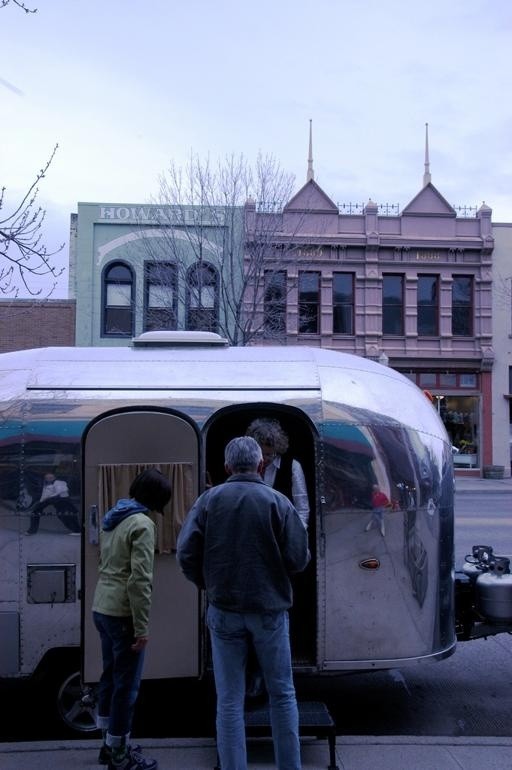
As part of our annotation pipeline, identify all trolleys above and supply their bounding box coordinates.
[397,479,431,606]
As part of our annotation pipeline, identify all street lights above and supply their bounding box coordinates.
[378,348,390,368]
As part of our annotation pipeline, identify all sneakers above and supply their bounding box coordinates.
[98,738,159,770]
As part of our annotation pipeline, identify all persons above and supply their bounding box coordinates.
[246,418,311,534]
[176,435,324,770]
[26,472,82,537]
[365,485,389,538]
[92,467,173,770]
